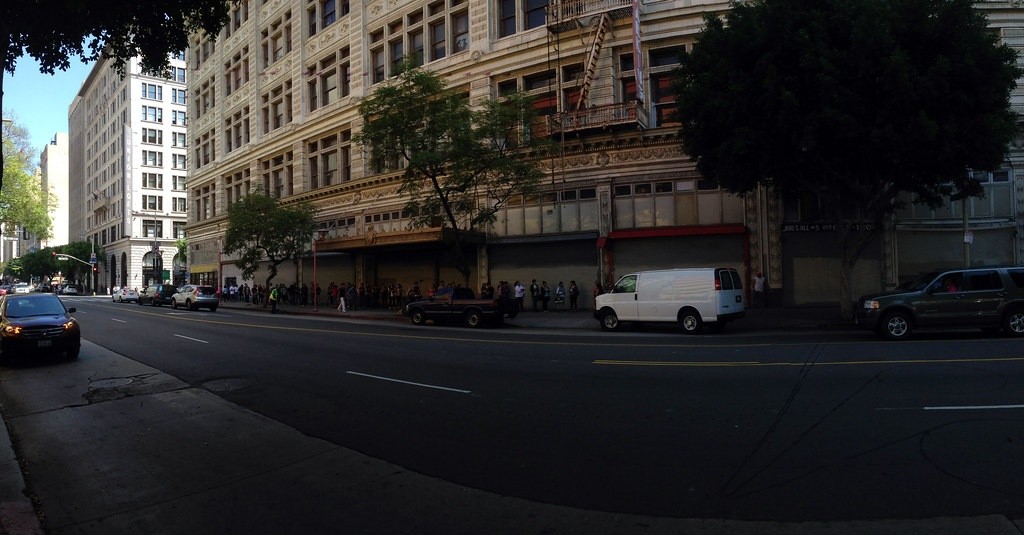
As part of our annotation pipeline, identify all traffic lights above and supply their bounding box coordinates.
[93,267,97,275]
[51,252,56,262]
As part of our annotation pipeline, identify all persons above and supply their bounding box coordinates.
[591,281,600,298]
[945,278,958,292]
[215,279,579,314]
[752,272,769,309]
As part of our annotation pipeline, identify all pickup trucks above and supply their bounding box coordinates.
[406,286,503,328]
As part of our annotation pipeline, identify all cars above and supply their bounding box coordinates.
[111,288,139,303]
[0,293,80,359]
[0,281,79,294]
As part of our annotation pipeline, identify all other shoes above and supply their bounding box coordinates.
[343,310,346,312]
[337,309,341,312]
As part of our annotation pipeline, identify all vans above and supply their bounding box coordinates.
[594,267,744,333]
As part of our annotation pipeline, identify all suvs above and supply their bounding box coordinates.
[848,264,1024,339]
[170,284,220,311]
[138,284,179,306]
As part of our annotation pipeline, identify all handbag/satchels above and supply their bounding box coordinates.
[554,294,563,303]
